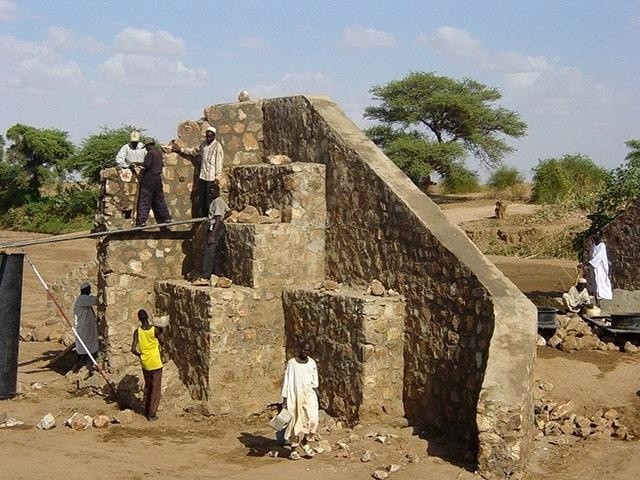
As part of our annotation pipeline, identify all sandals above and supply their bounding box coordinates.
[289,451,300,460]
[304,451,314,458]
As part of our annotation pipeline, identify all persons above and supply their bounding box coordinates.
[556,277,593,318]
[280,341,319,460]
[190,184,232,286]
[71,283,99,373]
[576,230,613,307]
[129,137,171,227]
[171,126,224,217]
[130,309,164,423]
[114,130,148,168]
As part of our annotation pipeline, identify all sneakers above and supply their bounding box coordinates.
[191,277,209,286]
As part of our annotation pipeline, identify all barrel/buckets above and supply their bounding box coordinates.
[587,306,601,316]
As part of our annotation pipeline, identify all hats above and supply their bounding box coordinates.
[577,277,589,283]
[129,131,139,142]
[80,282,91,290]
[205,126,217,135]
[141,137,155,150]
